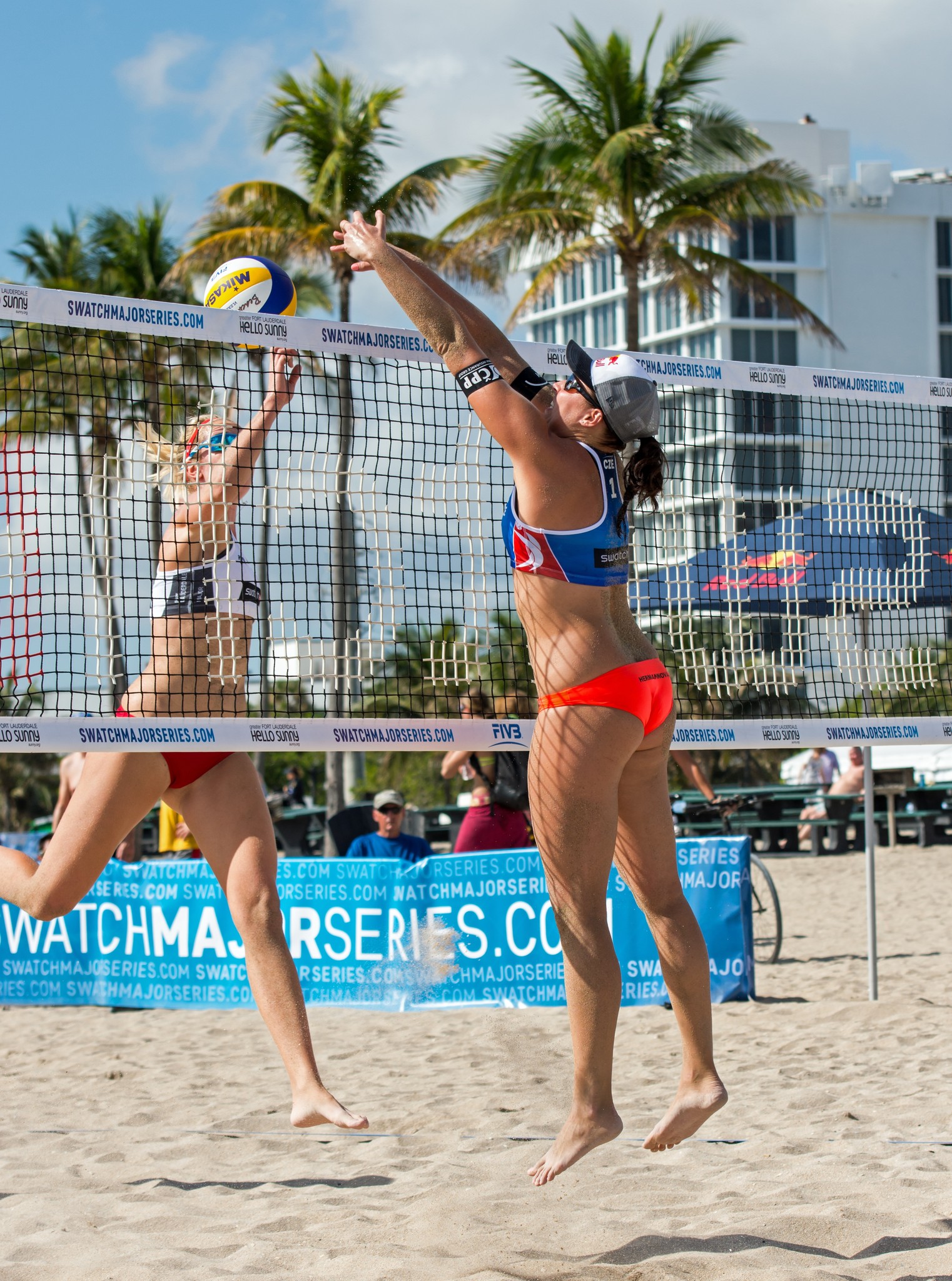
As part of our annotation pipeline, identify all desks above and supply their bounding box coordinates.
[764,795,860,855]
[681,795,742,802]
[273,805,325,858]
[672,787,822,792]
[415,805,469,846]
[873,784,952,811]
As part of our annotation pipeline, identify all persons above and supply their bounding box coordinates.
[670,750,737,818]
[37,833,54,860]
[0,347,367,1130]
[330,207,727,1188]
[782,745,866,850]
[158,799,203,860]
[441,686,539,854]
[279,764,435,864]
[52,712,136,862]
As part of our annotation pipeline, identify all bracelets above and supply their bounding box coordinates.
[710,796,717,804]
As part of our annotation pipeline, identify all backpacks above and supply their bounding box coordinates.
[467,751,529,817]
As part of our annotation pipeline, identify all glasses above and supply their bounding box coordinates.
[563,373,610,427]
[457,702,470,712]
[376,806,402,815]
[179,433,238,469]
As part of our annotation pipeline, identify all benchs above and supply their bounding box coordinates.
[681,810,947,848]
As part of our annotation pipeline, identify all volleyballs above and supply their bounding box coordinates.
[203,255,298,350]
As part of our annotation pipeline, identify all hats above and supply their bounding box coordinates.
[566,339,660,444]
[372,789,404,809]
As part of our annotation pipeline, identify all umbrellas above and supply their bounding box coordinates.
[627,488,952,1004]
